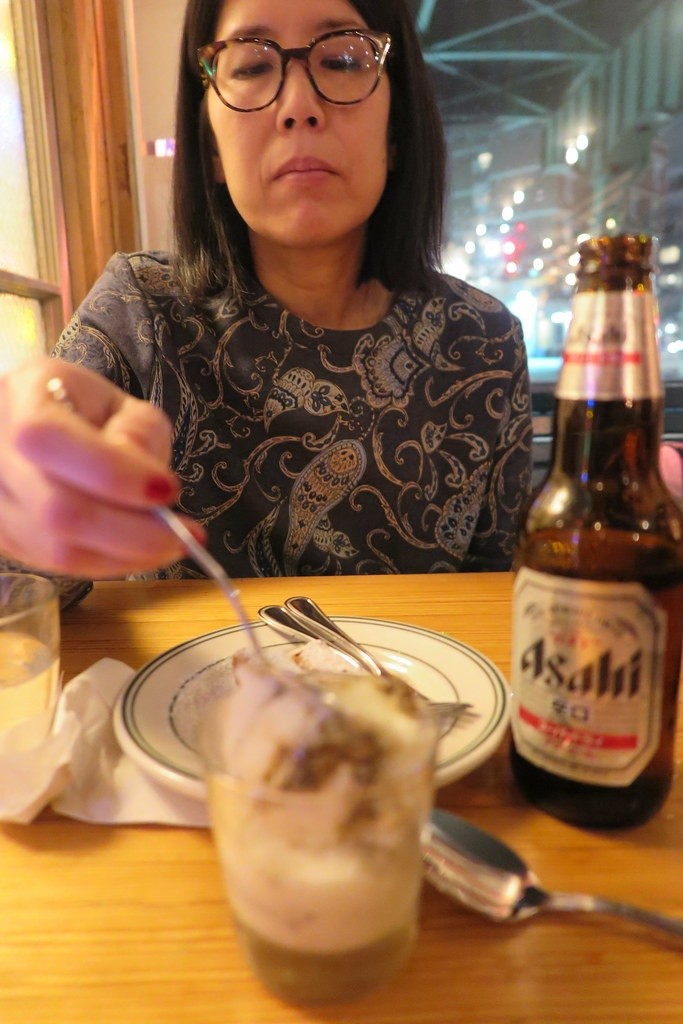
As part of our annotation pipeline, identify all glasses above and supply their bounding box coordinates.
[197,29,391,113]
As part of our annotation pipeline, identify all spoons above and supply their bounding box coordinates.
[418,807,683,938]
[48,376,355,733]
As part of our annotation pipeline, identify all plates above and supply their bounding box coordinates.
[111,617,511,800]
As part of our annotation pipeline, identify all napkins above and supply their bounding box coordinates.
[1,655,211,831]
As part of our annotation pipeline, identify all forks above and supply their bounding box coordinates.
[258,596,472,725]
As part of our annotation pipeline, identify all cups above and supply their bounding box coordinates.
[199,669,439,1011]
[0,572,61,751]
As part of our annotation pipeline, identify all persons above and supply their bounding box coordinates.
[0,1,532,613]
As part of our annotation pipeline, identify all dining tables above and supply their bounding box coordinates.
[1,570,683,1024]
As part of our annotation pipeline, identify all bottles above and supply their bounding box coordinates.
[506,229,683,834]
